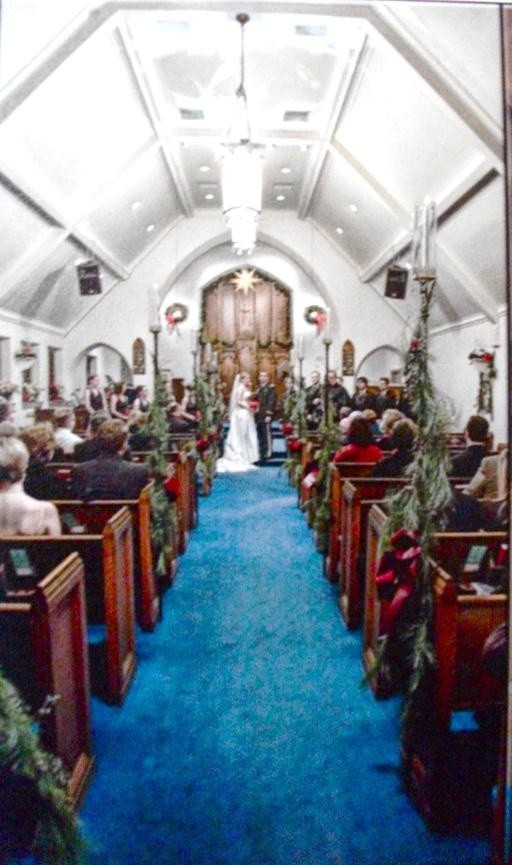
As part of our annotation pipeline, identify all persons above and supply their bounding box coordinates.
[253,371,276,459]
[213,372,260,473]
[1,436,61,535]
[283,371,507,534]
[1,370,227,464]
[18,428,72,500]
[70,419,149,500]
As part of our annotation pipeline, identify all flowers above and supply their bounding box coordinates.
[247,401,260,410]
[303,305,328,326]
[468,342,500,423]
[165,303,188,323]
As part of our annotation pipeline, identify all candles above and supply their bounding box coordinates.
[150,284,165,326]
[416,199,435,265]
[325,302,334,338]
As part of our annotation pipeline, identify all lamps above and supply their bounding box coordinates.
[209,13,271,257]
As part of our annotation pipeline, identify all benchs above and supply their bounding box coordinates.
[278,416,509,831]
[0,388,229,835]
